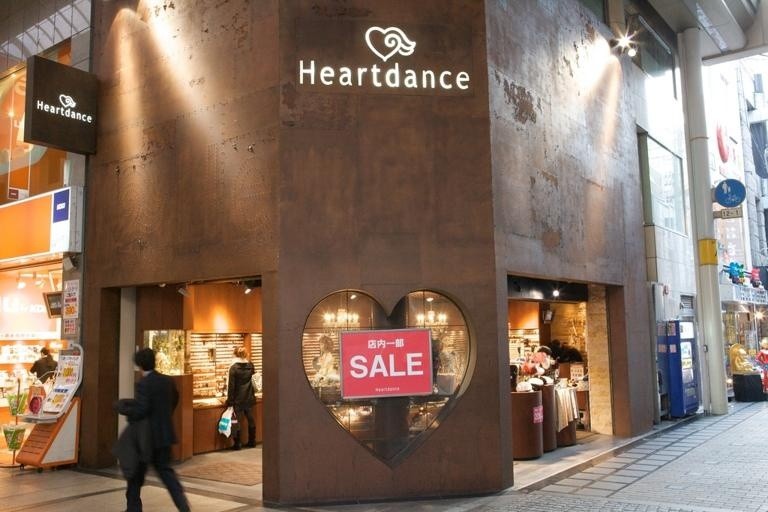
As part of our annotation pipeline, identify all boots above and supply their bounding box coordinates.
[232,429,241,450]
[242,426,256,448]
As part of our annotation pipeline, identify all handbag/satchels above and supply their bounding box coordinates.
[231,412,240,431]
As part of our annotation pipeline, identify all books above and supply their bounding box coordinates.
[39,383,77,414]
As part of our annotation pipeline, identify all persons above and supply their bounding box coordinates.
[756,336,768,393]
[309,333,340,386]
[734,348,756,373]
[108,345,198,512]
[436,332,461,372]
[29,395,43,414]
[223,344,260,451]
[28,346,59,384]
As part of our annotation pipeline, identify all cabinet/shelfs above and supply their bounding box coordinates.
[531,383,555,453]
[510,390,543,461]
[555,385,577,448]
[0,338,60,432]
[192,391,263,456]
